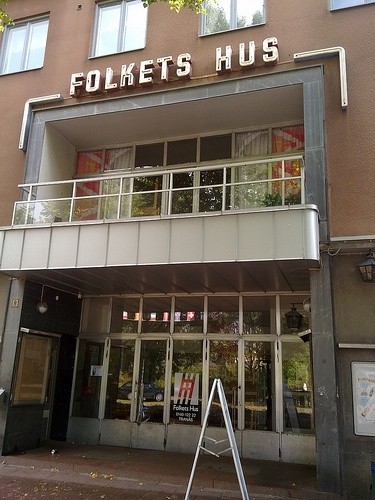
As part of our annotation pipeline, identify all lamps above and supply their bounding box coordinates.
[355,248,375,283]
[284,303,303,330]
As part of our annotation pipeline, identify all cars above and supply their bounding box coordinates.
[116,383,165,402]
